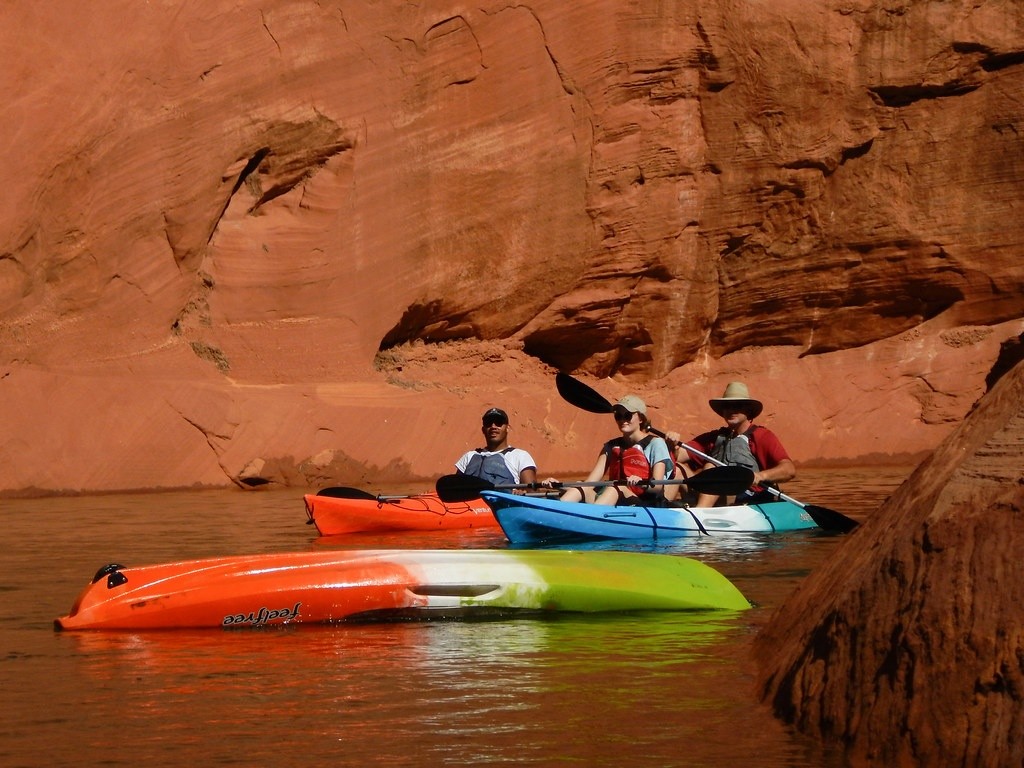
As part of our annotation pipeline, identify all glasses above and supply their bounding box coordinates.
[614,411,636,422]
[483,416,508,427]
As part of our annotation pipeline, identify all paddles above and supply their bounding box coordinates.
[436,465,755,503]
[316,486,527,500]
[555,372,860,534]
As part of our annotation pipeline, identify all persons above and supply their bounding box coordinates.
[665,382,796,508]
[540,395,674,506]
[455,408,538,496]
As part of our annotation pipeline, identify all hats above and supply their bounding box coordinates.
[612,394,647,413]
[709,381,763,420]
[482,407,508,423]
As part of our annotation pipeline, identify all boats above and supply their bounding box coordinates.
[299,493,530,536]
[54,550,756,627]
[478,489,821,545]
